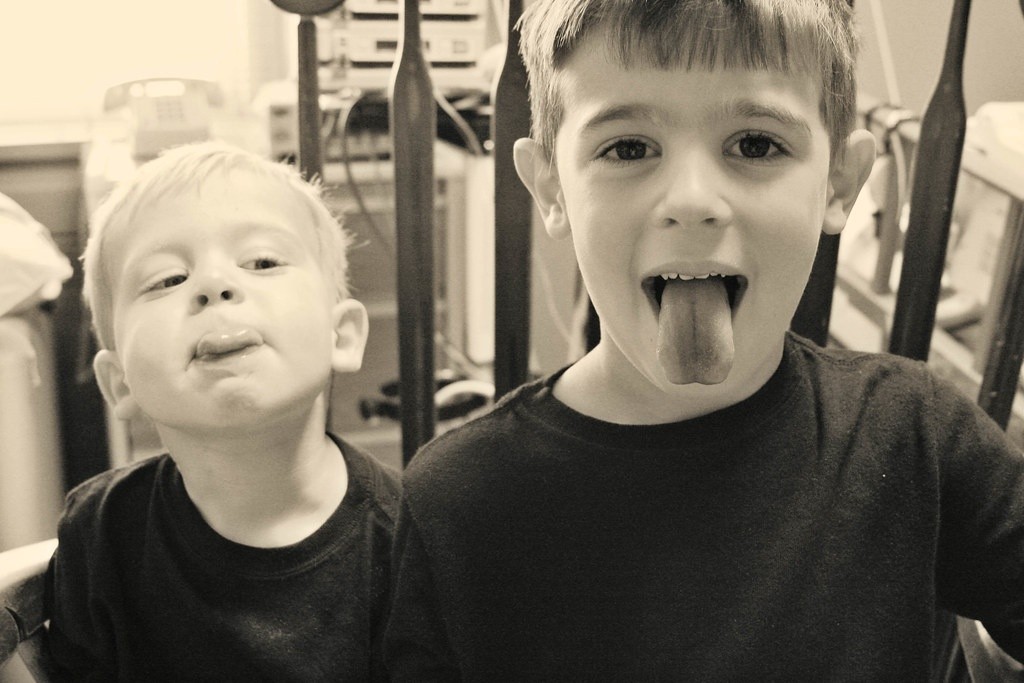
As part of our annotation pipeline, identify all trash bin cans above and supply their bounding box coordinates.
[355,368,498,470]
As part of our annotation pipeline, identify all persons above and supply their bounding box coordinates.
[44,146,403,682]
[380,0,1023,683]
